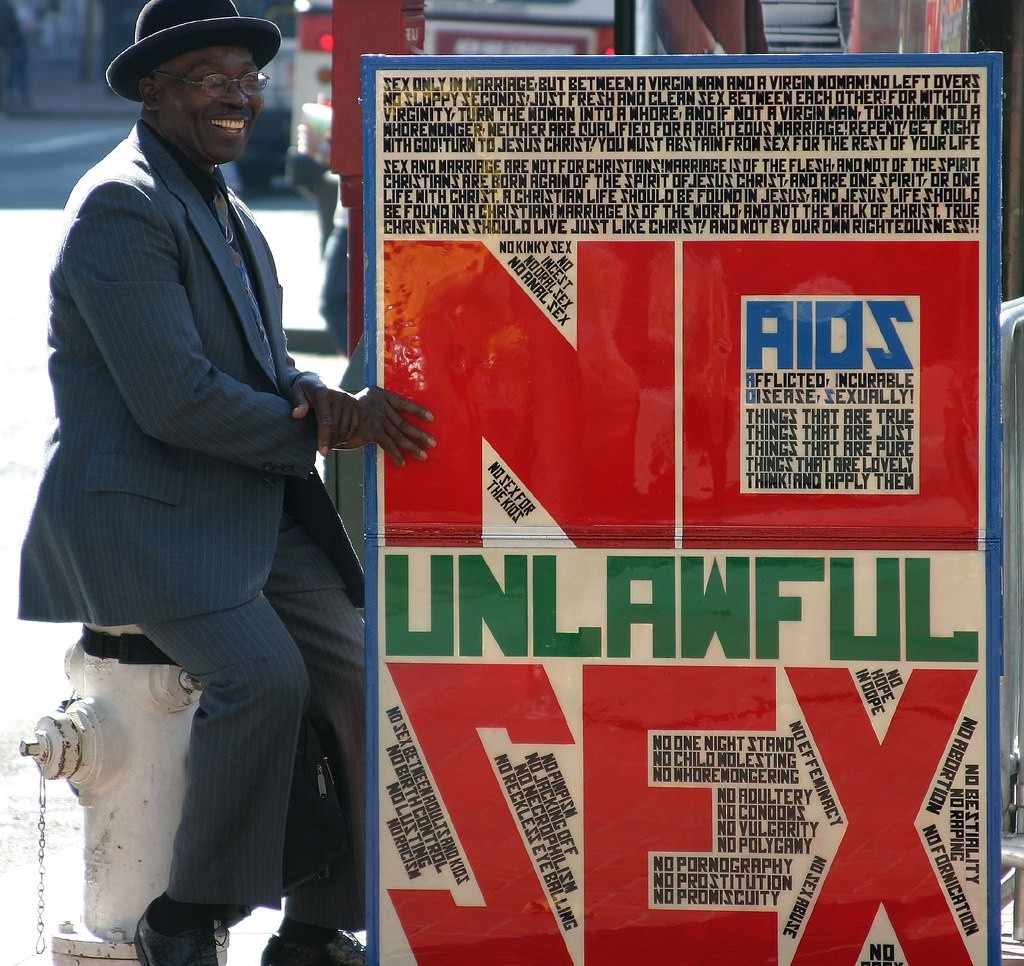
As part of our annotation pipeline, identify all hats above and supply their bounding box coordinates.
[106,0,282,103]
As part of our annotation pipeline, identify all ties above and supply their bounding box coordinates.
[213,184,276,381]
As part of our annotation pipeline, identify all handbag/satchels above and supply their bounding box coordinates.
[214,712,350,935]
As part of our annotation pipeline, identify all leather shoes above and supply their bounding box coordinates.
[134,896,229,966]
[261,932,365,966]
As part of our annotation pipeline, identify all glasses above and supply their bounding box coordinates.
[153,72,270,98]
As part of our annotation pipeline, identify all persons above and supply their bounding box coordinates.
[18,0,436,966]
